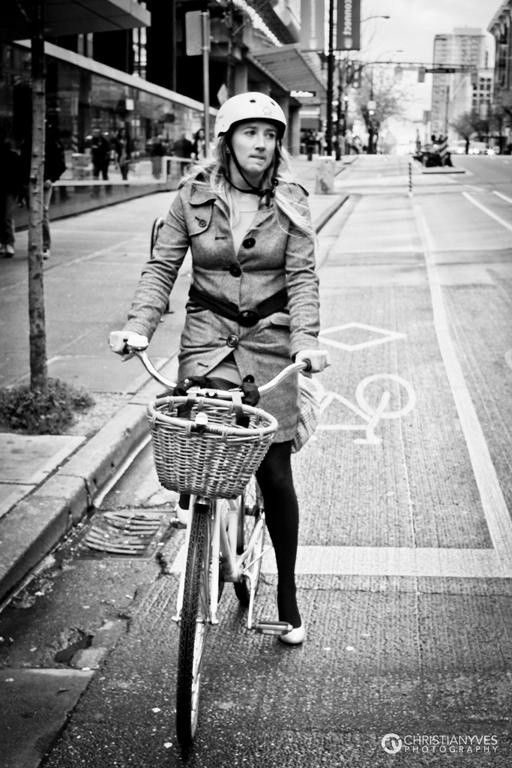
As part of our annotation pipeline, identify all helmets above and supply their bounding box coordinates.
[213,90,287,201]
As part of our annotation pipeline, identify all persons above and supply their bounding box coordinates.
[106,92,331,648]
[303,125,352,160]
[0,112,206,262]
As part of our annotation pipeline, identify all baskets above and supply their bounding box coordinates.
[145,393,280,503]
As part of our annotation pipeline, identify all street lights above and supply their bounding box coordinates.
[369,46,405,153]
[336,13,391,160]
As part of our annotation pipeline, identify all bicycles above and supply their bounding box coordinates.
[113,337,313,748]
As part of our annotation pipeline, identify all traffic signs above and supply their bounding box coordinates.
[424,67,456,74]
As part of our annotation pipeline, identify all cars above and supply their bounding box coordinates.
[449,141,482,154]
[146,136,174,153]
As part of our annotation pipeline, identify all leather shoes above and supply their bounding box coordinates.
[279,618,305,646]
